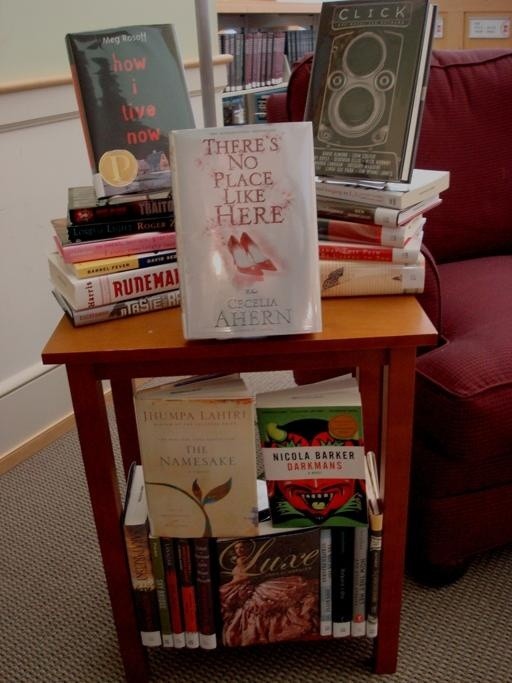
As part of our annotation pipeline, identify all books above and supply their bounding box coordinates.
[170,121,323,340]
[254,374,370,529]
[306,2,438,182]
[123,450,382,648]
[220,28,318,124]
[314,171,451,296]
[51,187,182,328]
[132,371,259,538]
[66,25,195,199]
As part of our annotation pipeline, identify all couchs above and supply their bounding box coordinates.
[264,46,510,592]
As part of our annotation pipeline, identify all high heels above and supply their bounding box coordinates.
[240,232,276,271]
[228,235,263,276]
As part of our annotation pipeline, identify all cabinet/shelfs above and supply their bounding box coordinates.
[41,297,440,682]
[218,0,512,125]
